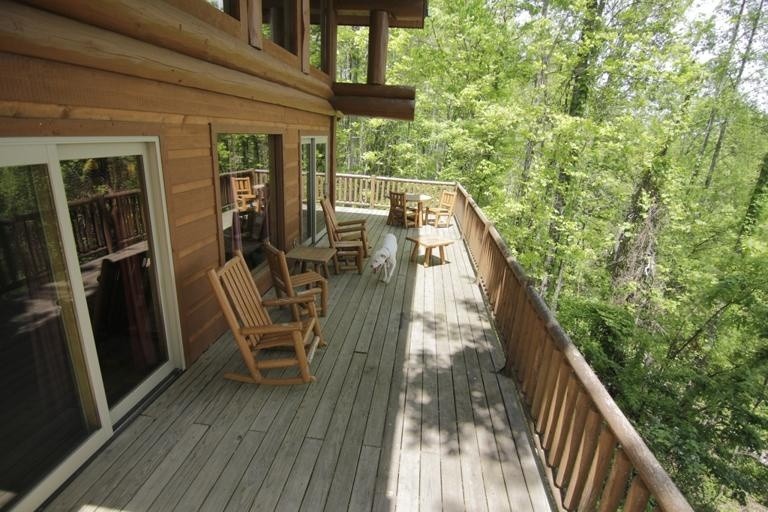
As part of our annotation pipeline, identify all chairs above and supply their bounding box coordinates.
[207,196,373,385]
[386,190,457,229]
[230,175,260,213]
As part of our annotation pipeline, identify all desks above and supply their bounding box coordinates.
[405,233,454,268]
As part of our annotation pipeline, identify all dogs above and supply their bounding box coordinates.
[370,233,398,284]
